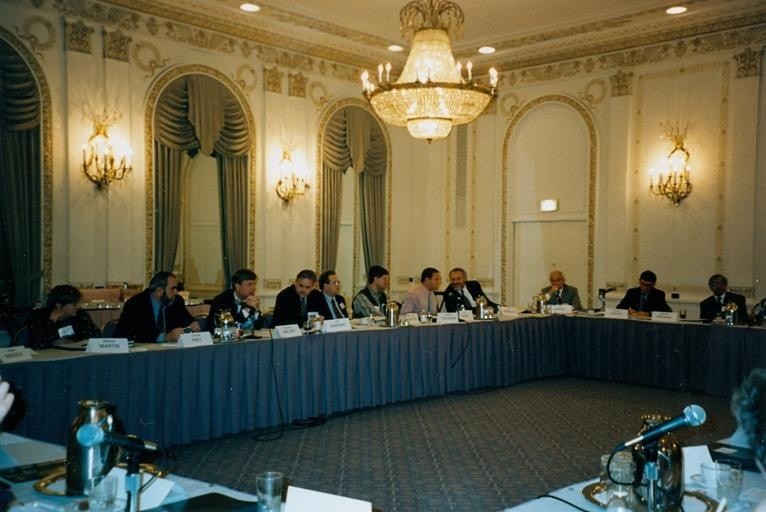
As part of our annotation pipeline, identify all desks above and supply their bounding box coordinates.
[0,304,554,465]
[554,309,766,399]
[0,424,294,512]
[494,443,766,511]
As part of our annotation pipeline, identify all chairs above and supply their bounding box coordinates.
[196,313,207,332]
[8,324,35,350]
[261,312,275,328]
[100,318,120,338]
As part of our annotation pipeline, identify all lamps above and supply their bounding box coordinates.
[647,140,695,208]
[273,152,313,204]
[76,121,134,192]
[359,0,500,144]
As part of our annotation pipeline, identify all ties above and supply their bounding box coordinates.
[156,305,165,333]
[461,290,472,309]
[427,294,432,313]
[301,297,307,317]
[557,290,722,307]
[330,299,343,318]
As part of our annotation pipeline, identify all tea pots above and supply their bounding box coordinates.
[631,415,685,511]
[383,299,402,326]
[475,294,487,318]
[65,399,124,495]
[213,305,236,328]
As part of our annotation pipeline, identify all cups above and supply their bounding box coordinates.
[726,309,734,325]
[702,462,732,485]
[210,328,244,343]
[537,300,544,314]
[482,309,494,319]
[599,451,635,511]
[680,309,687,318]
[258,480,290,501]
[86,475,118,512]
[715,459,742,505]
[255,472,283,511]
[418,311,438,324]
[368,314,375,326]
[303,316,324,331]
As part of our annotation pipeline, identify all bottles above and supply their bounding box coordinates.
[460,305,465,311]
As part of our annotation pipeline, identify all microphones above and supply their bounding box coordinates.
[451,283,464,299]
[233,298,257,313]
[74,422,162,458]
[625,402,708,452]
[607,284,625,292]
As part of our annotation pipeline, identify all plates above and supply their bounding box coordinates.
[33,461,171,498]
[692,473,717,490]
[581,480,729,512]
